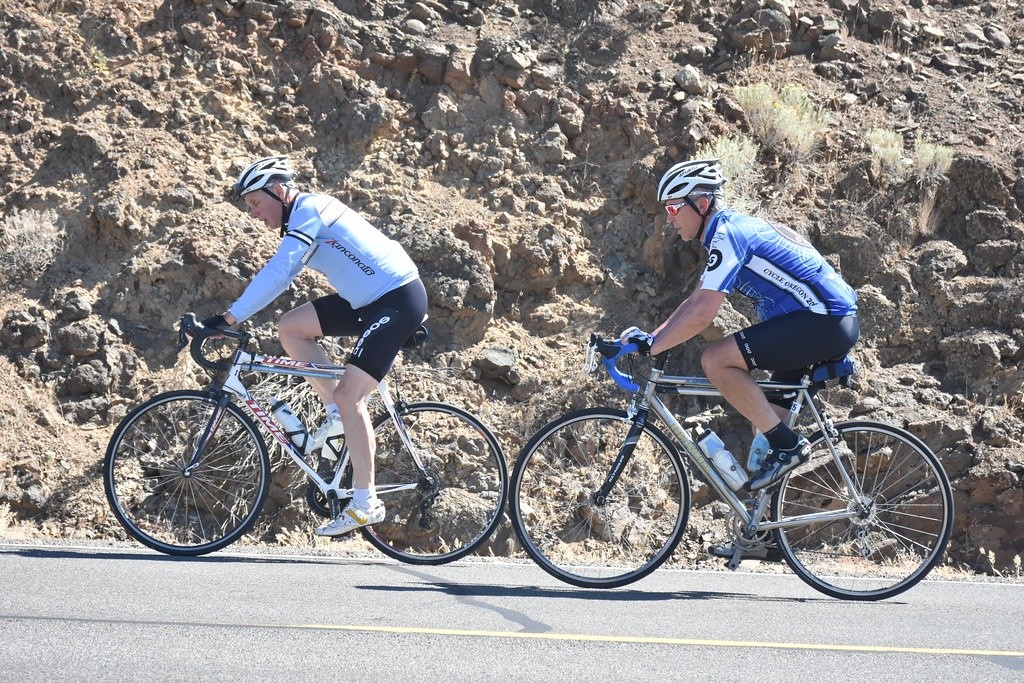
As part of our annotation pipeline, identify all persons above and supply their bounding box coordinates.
[201,158,428,538]
[620,160,861,562]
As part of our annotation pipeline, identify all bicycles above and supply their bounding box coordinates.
[102,312,508,565]
[508,330,956,601]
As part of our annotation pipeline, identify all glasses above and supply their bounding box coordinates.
[664,202,688,215]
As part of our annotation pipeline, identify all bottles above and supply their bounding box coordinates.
[746,429,772,473]
[322,415,345,461]
[267,397,317,455]
[695,425,750,493]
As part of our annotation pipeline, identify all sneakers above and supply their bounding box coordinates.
[751,433,811,490]
[708,541,777,557]
[315,499,386,536]
[311,409,345,453]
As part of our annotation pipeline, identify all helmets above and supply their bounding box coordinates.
[656,158,726,202]
[233,154,293,197]
